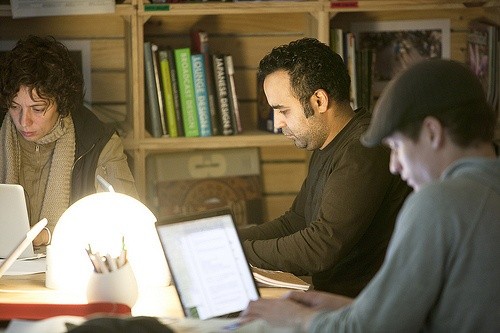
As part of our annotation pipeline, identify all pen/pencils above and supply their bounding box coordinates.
[85,236,127,273]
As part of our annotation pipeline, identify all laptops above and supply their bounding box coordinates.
[155,206,263,330]
[0,183,46,260]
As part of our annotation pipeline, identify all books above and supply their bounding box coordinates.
[331,27,376,115]
[145,31,242,138]
[254,70,283,134]
[468,20,500,113]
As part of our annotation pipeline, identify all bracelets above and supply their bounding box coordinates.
[43,226,52,246]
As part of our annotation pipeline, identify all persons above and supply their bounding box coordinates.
[236,37,415,300]
[0,35,140,248]
[238,57,500,332]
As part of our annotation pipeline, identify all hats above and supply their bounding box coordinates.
[358,58,487,149]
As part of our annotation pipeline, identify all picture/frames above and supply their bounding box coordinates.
[0,40,92,107]
[351,18,450,91]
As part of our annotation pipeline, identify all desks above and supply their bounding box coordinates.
[0,273,306,333]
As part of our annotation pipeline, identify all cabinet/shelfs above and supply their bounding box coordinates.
[0,0,500,224]
